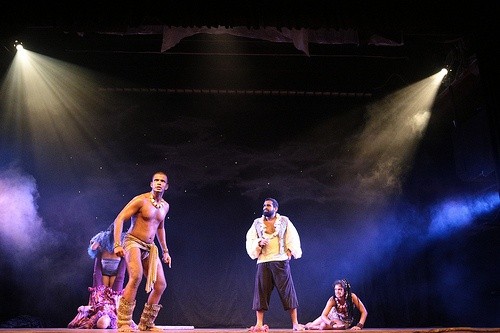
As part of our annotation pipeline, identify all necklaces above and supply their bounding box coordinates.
[151,194,164,208]
[335,298,346,308]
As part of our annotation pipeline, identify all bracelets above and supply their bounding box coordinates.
[114,243,121,248]
[162,249,168,253]
[357,323,362,329]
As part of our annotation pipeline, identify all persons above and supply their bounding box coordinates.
[113,171,171,333]
[245,197,306,331]
[88,223,127,291]
[70,286,137,329]
[300,278,367,330]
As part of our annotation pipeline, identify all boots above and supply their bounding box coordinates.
[137,303,163,331]
[118,297,137,333]
